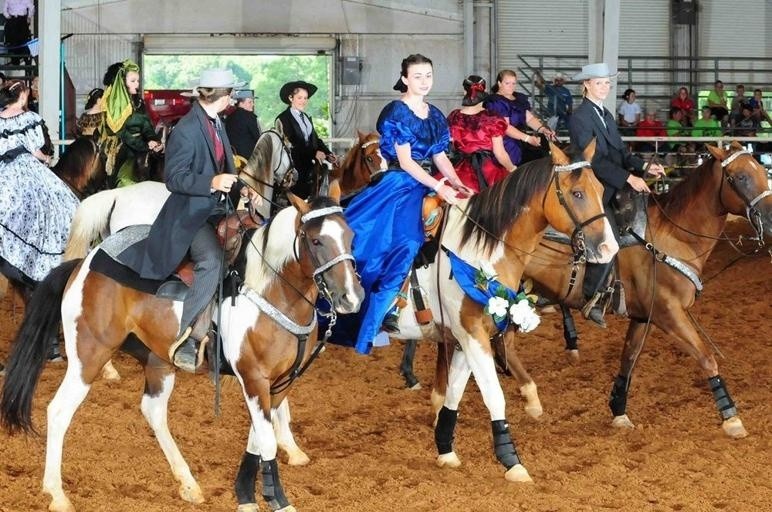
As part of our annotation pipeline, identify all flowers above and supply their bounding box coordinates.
[476,269,541,336]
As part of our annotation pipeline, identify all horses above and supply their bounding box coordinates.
[265,128,621,483]
[1,125,366,512]
[430,140,772,439]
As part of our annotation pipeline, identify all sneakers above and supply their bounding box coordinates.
[579,298,606,328]
[174,340,196,374]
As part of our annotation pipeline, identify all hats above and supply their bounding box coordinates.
[571,62,621,81]
[194,68,259,99]
[280,81,317,104]
